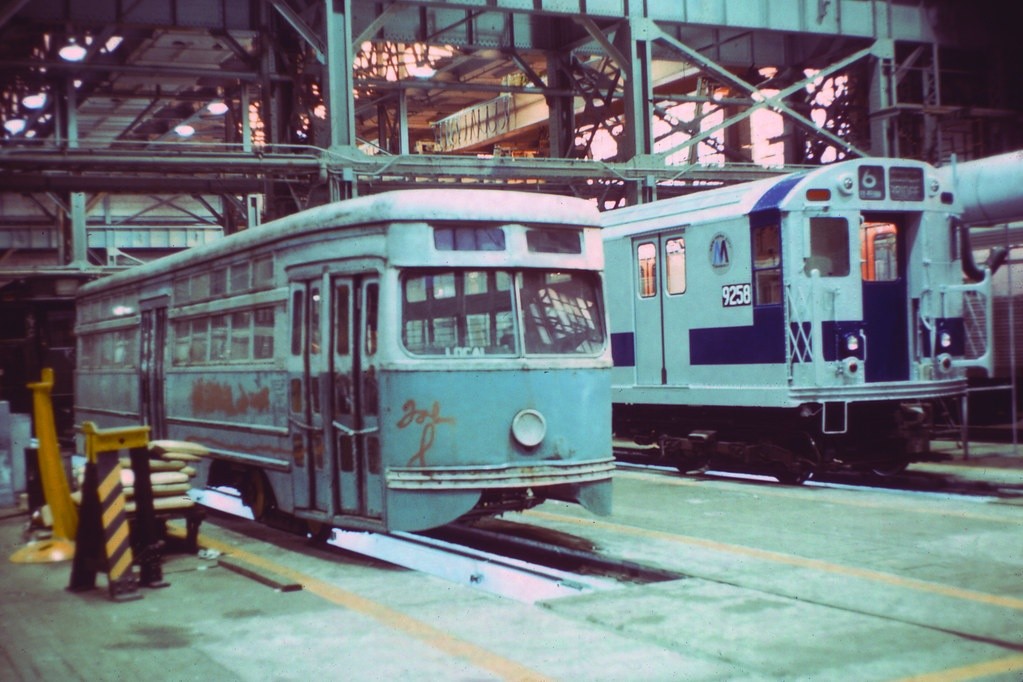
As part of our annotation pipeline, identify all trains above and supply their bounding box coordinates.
[936,149,1022,442]
[66,186,619,546]
[549,155,996,488]
